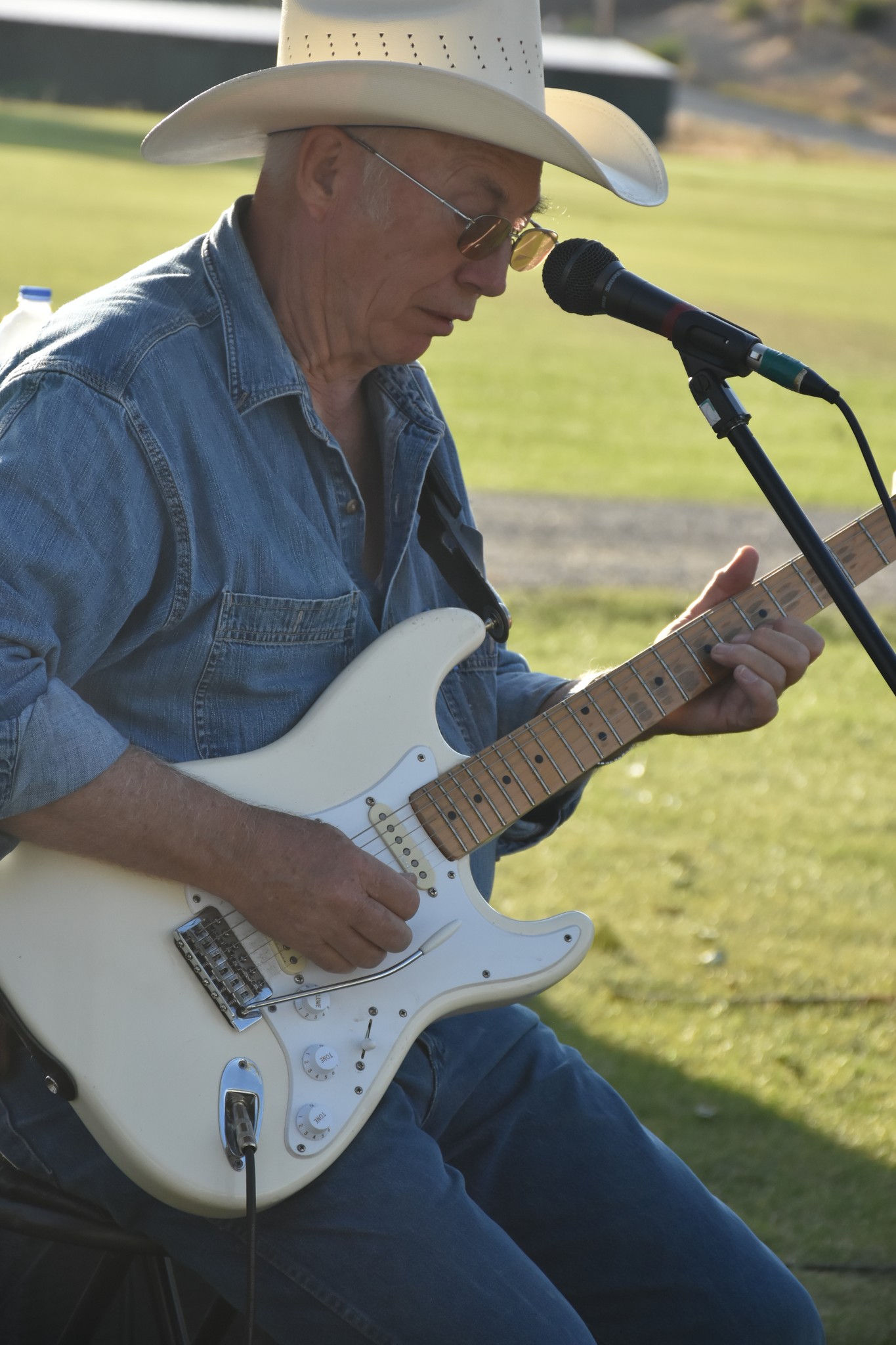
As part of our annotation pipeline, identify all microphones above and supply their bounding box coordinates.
[541,237,840,405]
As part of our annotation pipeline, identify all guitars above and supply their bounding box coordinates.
[0,489,896,1226]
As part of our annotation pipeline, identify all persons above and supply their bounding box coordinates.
[1,1,896,1344]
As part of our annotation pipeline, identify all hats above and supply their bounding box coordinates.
[138,0,673,210]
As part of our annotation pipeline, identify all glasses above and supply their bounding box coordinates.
[334,125,557,272]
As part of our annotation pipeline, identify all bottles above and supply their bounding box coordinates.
[0,285,53,371]
